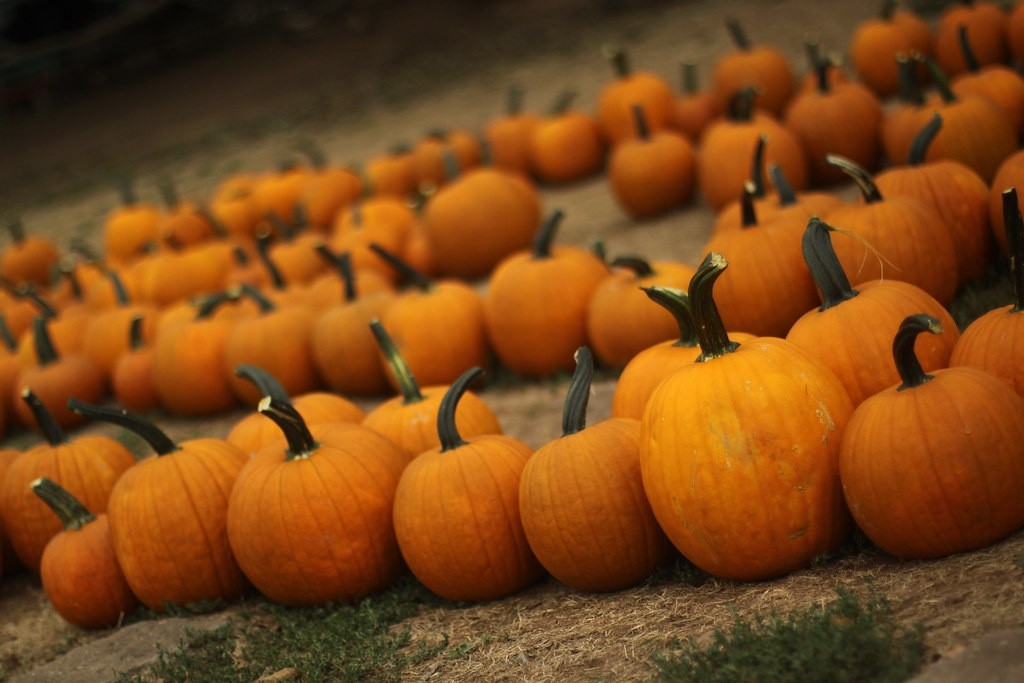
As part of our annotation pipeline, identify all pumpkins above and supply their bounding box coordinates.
[0,0,1024,632]
